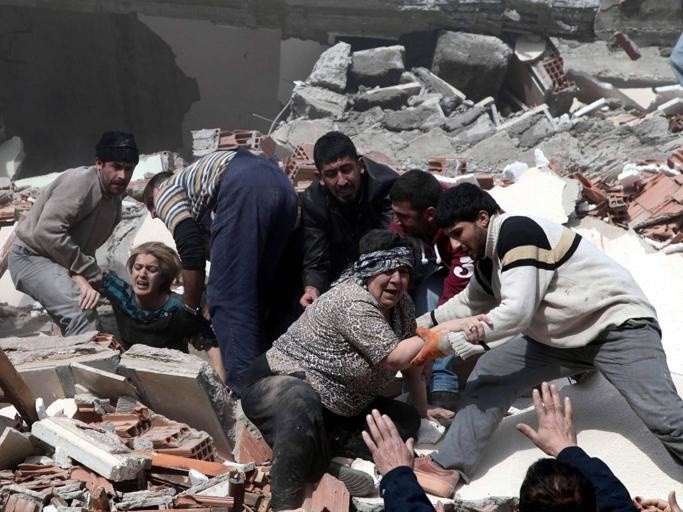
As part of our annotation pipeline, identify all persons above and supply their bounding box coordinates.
[8,131,299,397]
[238,129,682,512]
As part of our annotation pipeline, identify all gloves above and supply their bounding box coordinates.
[411,328,450,367]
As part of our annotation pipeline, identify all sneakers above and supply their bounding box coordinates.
[329,462,373,496]
[413,455,460,498]
